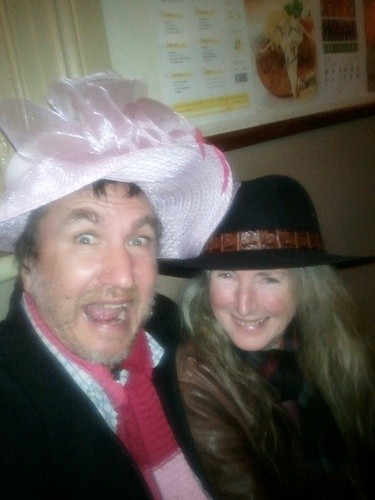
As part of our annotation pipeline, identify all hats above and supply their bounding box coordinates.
[155,175,374,276]
[1,73,237,258]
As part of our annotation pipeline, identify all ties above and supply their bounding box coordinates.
[18,291,209,500]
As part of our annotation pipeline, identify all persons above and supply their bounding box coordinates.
[0,69,235,500]
[156,175,375,500]
